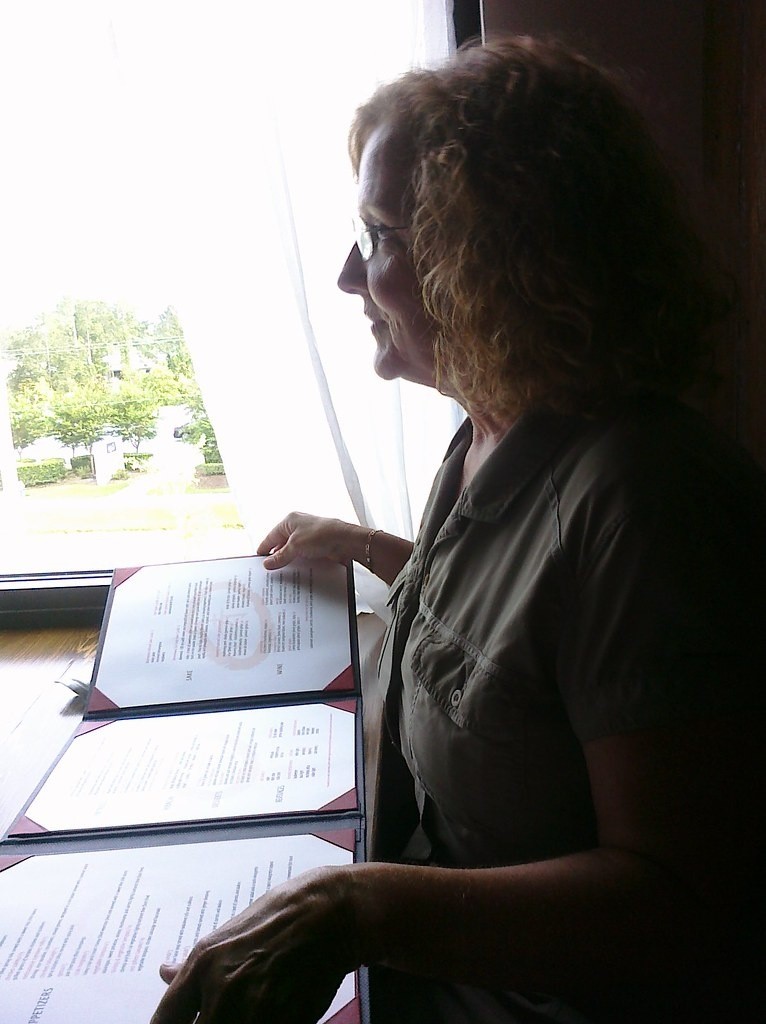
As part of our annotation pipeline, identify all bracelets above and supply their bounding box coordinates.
[365,529,384,574]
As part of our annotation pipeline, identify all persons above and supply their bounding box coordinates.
[152,31,766,1024]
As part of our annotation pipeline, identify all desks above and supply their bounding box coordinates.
[0,612,390,1024]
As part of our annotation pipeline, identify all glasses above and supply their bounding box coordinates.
[352,215,411,260]
